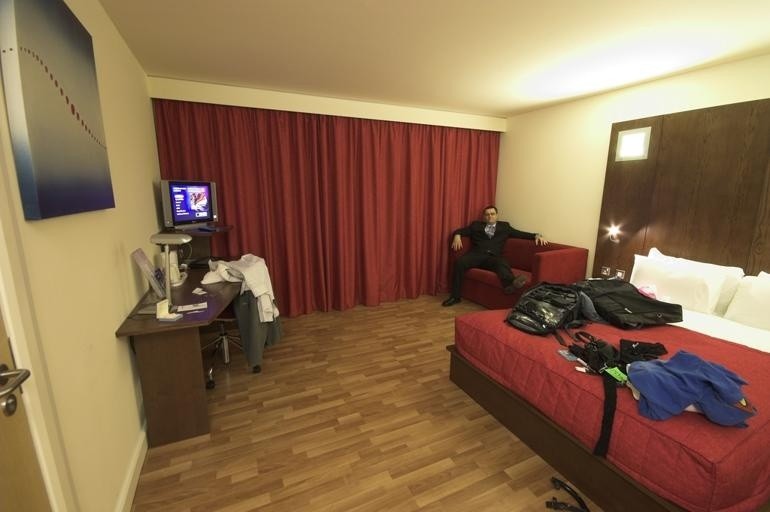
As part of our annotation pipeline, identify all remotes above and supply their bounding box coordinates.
[177,302,208,312]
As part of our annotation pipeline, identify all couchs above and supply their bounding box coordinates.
[450,235,590,310]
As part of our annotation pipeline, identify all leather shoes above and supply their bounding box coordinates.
[442,297,460,306]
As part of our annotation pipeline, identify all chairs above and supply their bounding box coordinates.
[198,257,269,391]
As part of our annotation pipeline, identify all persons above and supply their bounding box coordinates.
[442,205,549,306]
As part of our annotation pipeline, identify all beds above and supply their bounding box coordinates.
[445,248,770,512]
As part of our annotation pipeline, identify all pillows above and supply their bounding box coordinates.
[724,264,770,330]
[648,247,745,318]
[633,245,727,315]
[757,270,770,279]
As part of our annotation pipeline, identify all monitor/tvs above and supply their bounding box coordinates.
[159,179,219,230]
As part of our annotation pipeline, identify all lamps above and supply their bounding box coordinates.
[136,232,193,316]
[607,223,623,245]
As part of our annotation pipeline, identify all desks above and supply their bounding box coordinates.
[115,255,245,450]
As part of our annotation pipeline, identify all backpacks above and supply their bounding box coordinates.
[506,281,581,335]
